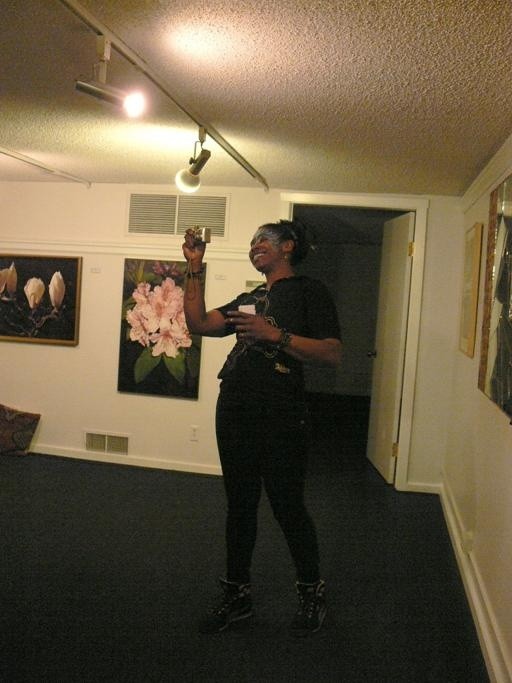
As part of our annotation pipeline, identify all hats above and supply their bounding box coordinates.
[280,220,309,265]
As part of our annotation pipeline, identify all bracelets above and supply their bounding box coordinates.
[186,267,206,279]
[276,328,292,349]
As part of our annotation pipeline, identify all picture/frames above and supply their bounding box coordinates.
[459,222,484,360]
[1,253,83,347]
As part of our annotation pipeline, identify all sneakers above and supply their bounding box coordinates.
[198,574,253,633]
[286,578,331,637]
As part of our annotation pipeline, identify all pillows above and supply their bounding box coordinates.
[0,403,41,458]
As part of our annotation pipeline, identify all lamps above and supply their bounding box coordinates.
[62,1,268,197]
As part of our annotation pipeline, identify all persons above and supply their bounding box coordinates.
[182,219,344,635]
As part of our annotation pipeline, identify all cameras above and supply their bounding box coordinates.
[192,224,211,243]
